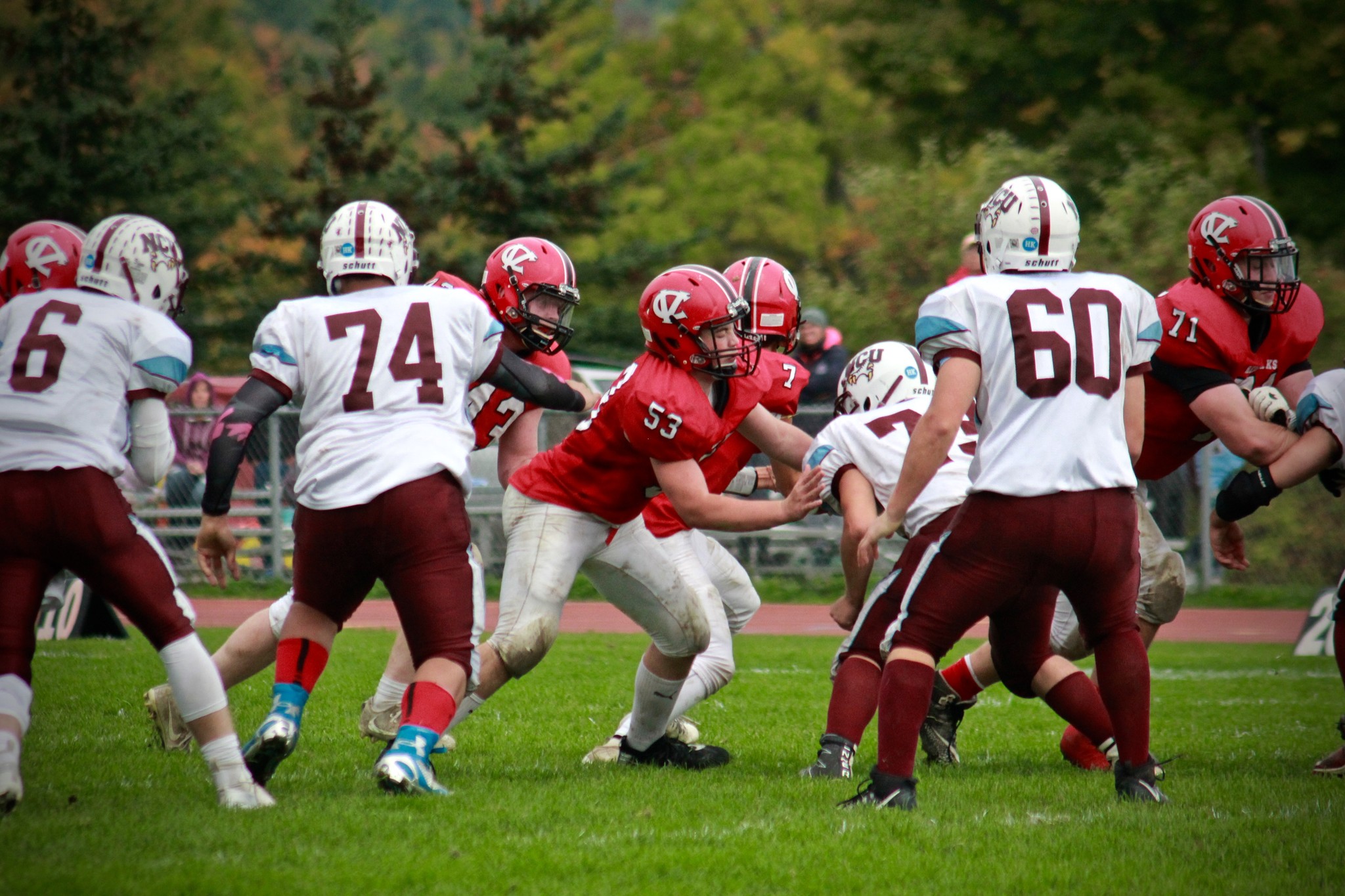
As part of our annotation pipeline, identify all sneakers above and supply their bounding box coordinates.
[218,783,276,811]
[580,745,622,764]
[1112,755,1169,805]
[358,699,457,753]
[1313,744,1345,772]
[143,681,193,756]
[835,765,918,811]
[917,668,978,771]
[616,730,729,771]
[0,758,23,818]
[663,710,702,744]
[239,711,298,787]
[1099,739,1166,781]
[797,733,858,781]
[374,744,452,795]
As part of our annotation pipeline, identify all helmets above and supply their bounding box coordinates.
[719,256,802,357]
[1188,195,1302,314]
[833,337,937,415]
[0,222,91,306]
[977,177,1082,278]
[638,262,749,380]
[318,199,418,294]
[75,213,188,319]
[479,236,582,355]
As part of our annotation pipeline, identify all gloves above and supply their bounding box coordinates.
[1249,386,1296,427]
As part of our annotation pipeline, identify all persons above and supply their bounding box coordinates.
[159,371,226,547]
[192,193,598,795]
[143,236,592,758]
[1,221,91,305]
[386,137,1345,808]
[0,214,278,811]
